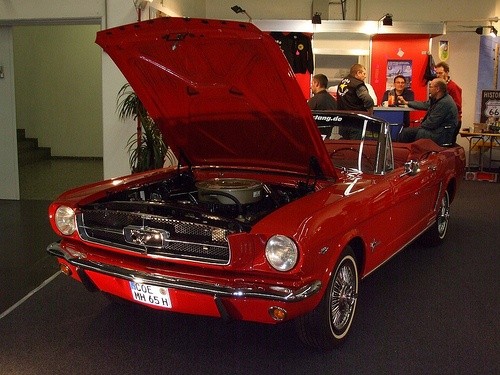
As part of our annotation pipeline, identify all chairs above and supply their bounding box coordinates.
[439,124,455,147]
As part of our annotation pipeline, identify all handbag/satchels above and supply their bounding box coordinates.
[424,55,437,80]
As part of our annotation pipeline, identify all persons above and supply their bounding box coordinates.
[382,76,414,107]
[335,63,374,140]
[398,78,458,146]
[308,74,337,139]
[435,62,463,129]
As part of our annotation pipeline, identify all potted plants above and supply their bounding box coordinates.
[115,81,176,175]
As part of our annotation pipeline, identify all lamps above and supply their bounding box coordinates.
[476,26,498,38]
[312,12,321,27]
[378,13,392,26]
[231,5,252,23]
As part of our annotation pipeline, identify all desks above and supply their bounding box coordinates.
[373,106,417,141]
[459,132,500,172]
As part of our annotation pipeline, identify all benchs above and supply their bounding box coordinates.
[324,140,409,173]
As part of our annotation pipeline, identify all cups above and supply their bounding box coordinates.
[382,101,388,107]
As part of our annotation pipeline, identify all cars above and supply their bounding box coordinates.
[47,16,467,351]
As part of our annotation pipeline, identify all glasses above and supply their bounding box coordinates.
[396,82,404,84]
